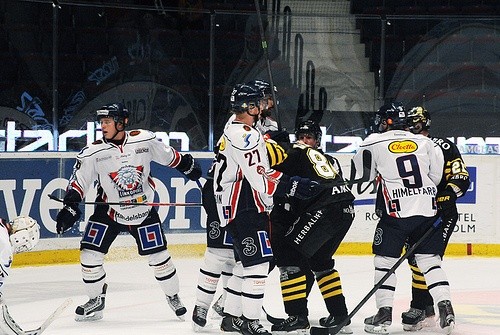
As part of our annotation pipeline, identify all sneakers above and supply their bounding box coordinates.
[193,305,208,331]
[319,317,353,333]
[401,308,426,331]
[364,307,392,334]
[438,300,456,334]
[239,319,272,335]
[271,315,310,333]
[75,282,107,320]
[220,315,241,331]
[212,294,227,317]
[166,293,187,321]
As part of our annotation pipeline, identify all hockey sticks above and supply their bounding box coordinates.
[253,0,283,133]
[38,297,74,335]
[319,149,373,188]
[310,217,443,335]
[48,194,205,207]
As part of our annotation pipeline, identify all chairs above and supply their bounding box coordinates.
[0,0,500,133]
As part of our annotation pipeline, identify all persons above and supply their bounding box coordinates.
[349,100,457,335]
[57,104,203,322]
[0,215,41,335]
[192,80,470,335]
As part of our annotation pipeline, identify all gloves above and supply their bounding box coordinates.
[287,175,321,199]
[56,202,82,234]
[176,153,202,180]
[435,185,456,209]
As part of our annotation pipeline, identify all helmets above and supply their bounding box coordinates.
[406,106,431,131]
[248,79,277,97]
[295,120,321,146]
[97,102,129,119]
[375,102,407,129]
[9,215,40,253]
[230,82,265,106]
